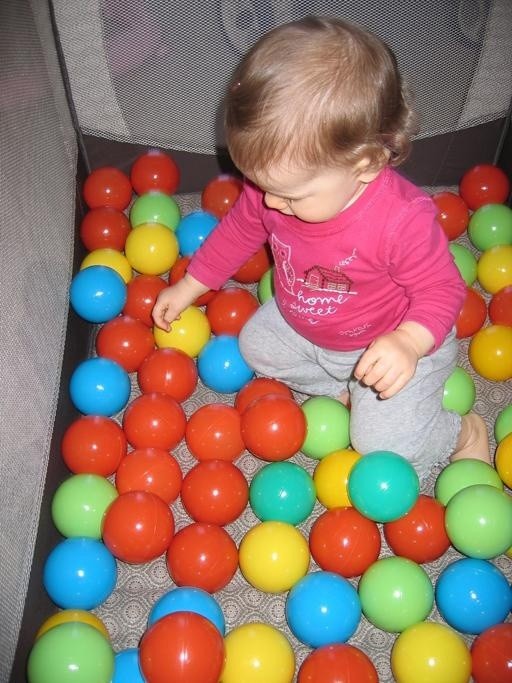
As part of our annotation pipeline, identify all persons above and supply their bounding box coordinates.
[151,13,494,464]
